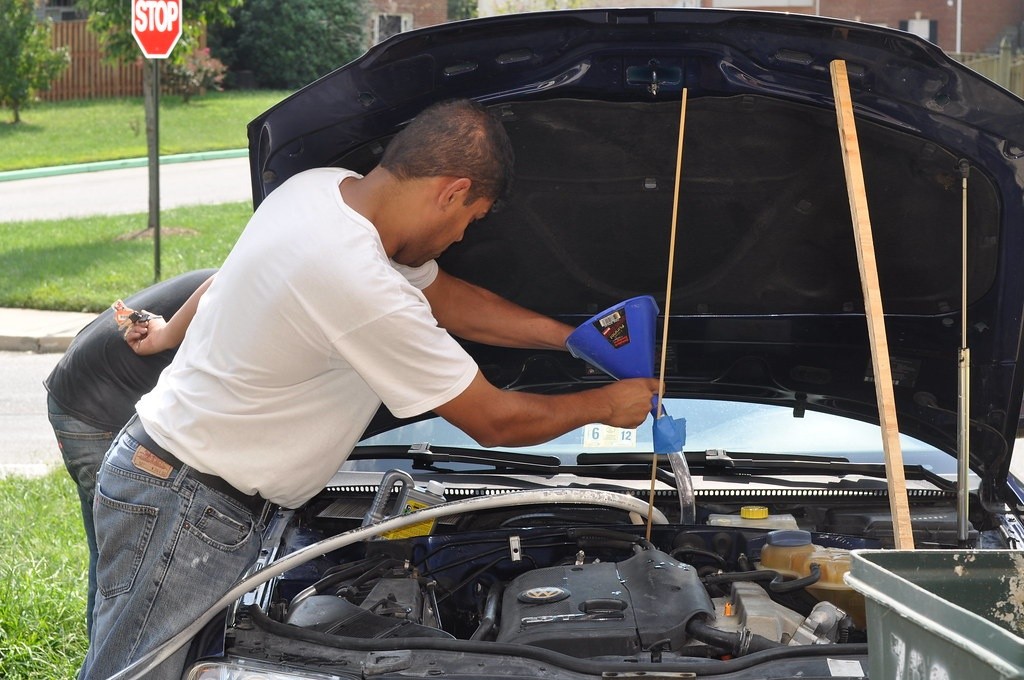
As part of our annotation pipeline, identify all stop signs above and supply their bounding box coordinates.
[131,0,183,61]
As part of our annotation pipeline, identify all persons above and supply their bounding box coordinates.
[43,267,218,644]
[74,99,665,680]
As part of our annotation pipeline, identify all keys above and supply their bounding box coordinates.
[118,311,139,331]
[139,314,162,322]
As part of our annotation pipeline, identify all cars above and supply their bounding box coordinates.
[184,9,1024,680]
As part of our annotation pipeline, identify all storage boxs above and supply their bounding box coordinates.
[843,549,1024,680]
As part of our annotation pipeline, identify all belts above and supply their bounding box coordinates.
[124,415,277,526]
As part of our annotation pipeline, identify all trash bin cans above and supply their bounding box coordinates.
[843,548,1024,680]
